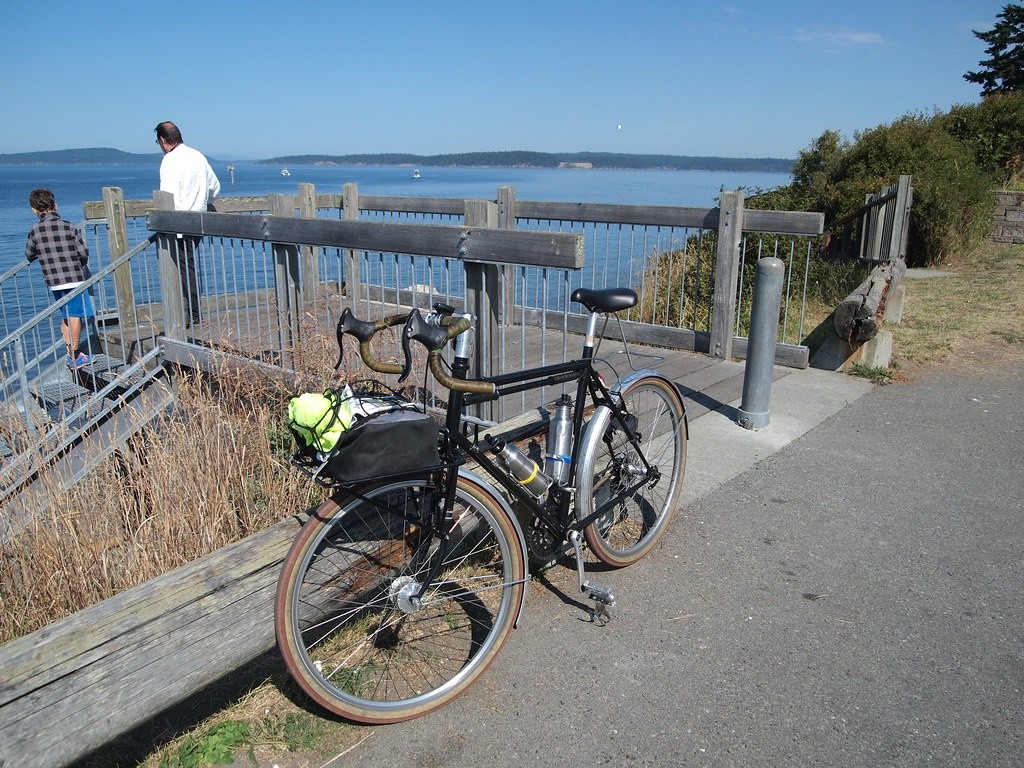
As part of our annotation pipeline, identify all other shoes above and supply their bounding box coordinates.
[186,317,203,324]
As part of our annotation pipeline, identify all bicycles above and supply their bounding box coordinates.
[273,286,690,727]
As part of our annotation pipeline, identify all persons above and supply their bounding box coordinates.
[154,121,220,327]
[24,190,97,371]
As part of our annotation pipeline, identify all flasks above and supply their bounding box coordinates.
[484,434,553,499]
[544,393,576,485]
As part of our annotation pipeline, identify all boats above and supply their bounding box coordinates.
[280,169,291,176]
[412,169,420,179]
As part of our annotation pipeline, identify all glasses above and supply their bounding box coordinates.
[155,139,160,145]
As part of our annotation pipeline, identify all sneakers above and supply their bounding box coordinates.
[66,352,96,370]
